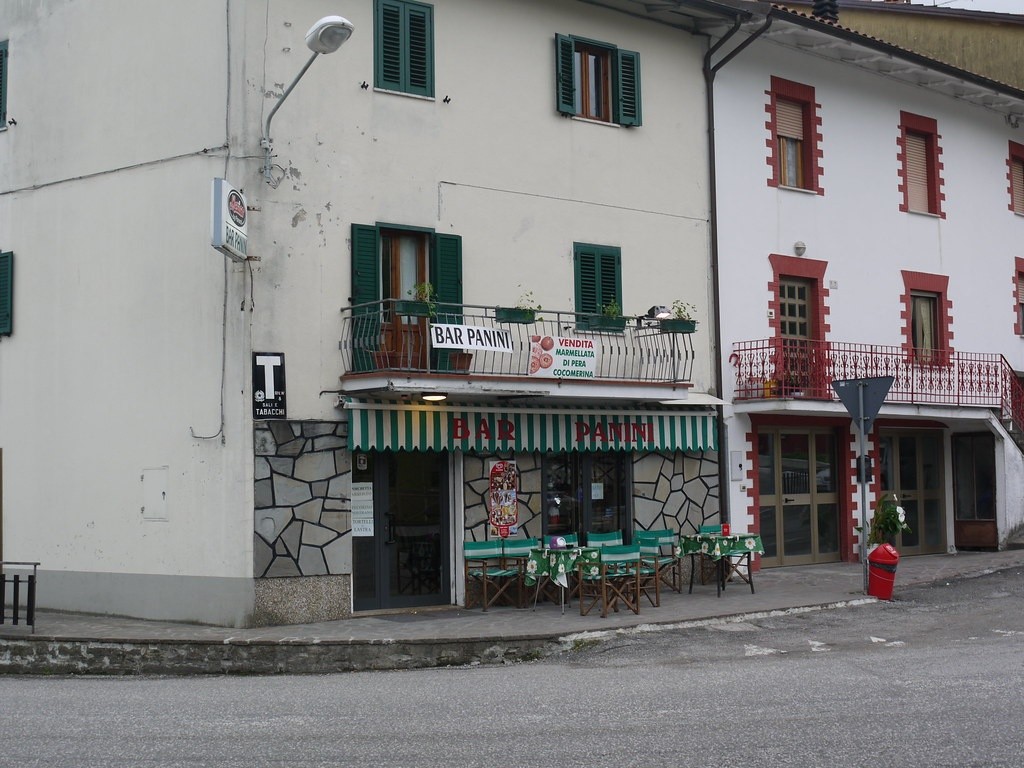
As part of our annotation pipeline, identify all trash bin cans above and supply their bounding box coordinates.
[868,542,899,600]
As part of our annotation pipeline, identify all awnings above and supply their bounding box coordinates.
[333,395,721,454]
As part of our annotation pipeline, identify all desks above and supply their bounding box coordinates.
[523,548,600,615]
[675,531,765,597]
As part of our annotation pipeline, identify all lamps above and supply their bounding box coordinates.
[422,392,448,401]
[644,306,665,319]
[262,15,356,184]
[794,242,807,257]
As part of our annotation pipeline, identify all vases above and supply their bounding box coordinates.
[764,380,797,396]
[450,353,473,370]
[371,323,420,368]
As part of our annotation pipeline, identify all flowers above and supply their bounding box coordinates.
[853,494,912,545]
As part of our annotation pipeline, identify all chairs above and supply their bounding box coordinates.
[576,541,641,618]
[614,536,658,607]
[587,529,636,606]
[462,541,524,613]
[634,529,682,595]
[699,525,734,586]
[501,538,560,608]
[543,531,594,608]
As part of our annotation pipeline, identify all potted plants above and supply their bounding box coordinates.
[495,287,544,324]
[587,293,638,330]
[394,281,439,318]
[658,299,699,332]
